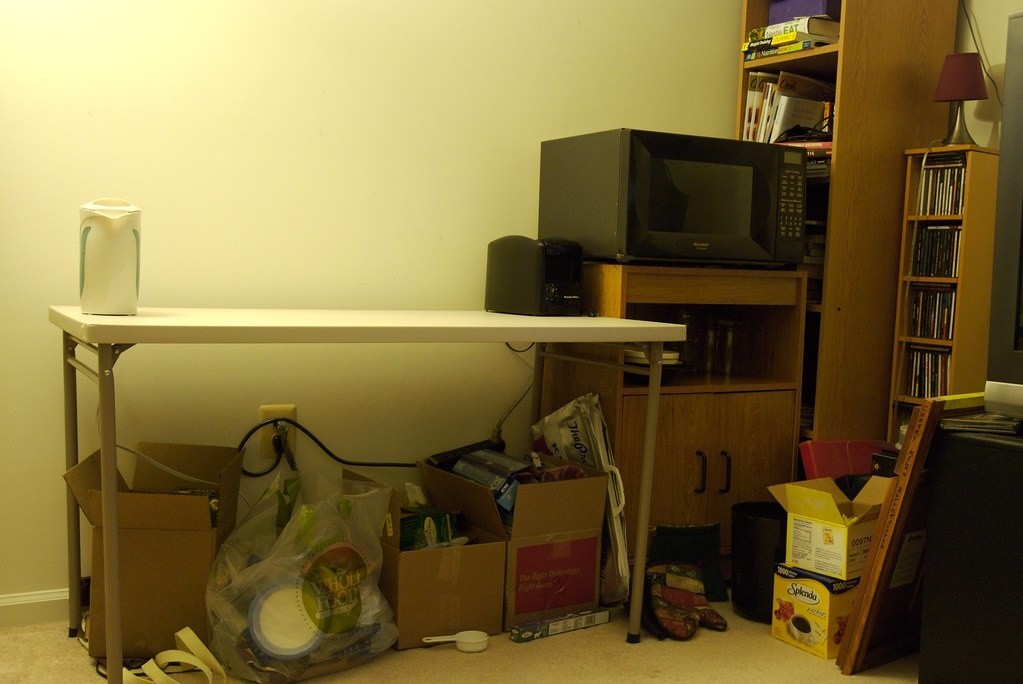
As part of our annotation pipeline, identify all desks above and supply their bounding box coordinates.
[47,305,685,684]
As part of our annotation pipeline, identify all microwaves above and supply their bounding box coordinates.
[538,130,806,269]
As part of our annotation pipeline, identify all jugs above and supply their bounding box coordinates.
[76,197,142,314]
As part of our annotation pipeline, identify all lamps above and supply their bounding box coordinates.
[931,48,988,147]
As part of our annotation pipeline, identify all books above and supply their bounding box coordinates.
[938,411,1022,438]
[904,154,970,396]
[741,15,841,144]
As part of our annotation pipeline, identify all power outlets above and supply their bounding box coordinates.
[257,404,296,460]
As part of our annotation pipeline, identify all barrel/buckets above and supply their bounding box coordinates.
[730,501,787,624]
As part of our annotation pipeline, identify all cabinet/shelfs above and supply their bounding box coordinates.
[881,140,1003,448]
[533,257,809,605]
[734,1,960,456]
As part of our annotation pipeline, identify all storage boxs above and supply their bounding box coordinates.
[60,442,248,662]
[503,454,610,637]
[762,472,897,660]
[336,457,508,655]
